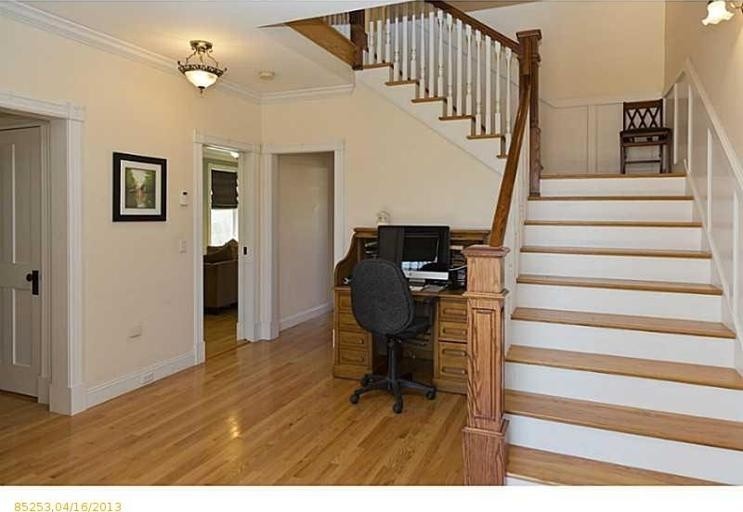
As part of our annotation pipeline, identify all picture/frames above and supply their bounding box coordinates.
[111,150,167,223]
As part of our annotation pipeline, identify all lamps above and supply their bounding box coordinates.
[699,0,743,28]
[175,40,229,97]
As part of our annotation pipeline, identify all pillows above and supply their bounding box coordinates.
[202,238,238,263]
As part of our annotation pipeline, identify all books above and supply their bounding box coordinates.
[405,284,445,293]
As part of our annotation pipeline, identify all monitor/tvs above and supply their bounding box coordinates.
[377,225,450,286]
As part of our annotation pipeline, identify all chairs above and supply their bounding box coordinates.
[346,256,436,414]
[203,239,238,316]
[618,99,674,175]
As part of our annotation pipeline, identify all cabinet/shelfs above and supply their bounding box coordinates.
[331,224,492,394]
